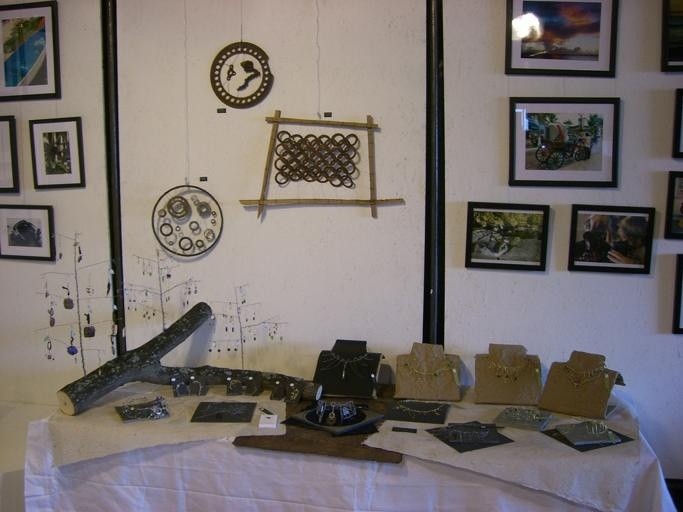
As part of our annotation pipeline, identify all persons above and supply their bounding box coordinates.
[578,133,591,148]
[576,132,585,152]
[582,214,647,265]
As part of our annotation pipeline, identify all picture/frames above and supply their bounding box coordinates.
[671,253,682,335]
[671,89,682,161]
[507,96,620,188]
[659,0,680,72]
[503,1,618,79]
[567,202,656,274]
[0,2,87,266]
[463,200,550,272]
[663,172,682,241]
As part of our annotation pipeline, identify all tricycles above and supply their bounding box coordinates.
[535,137,585,170]
